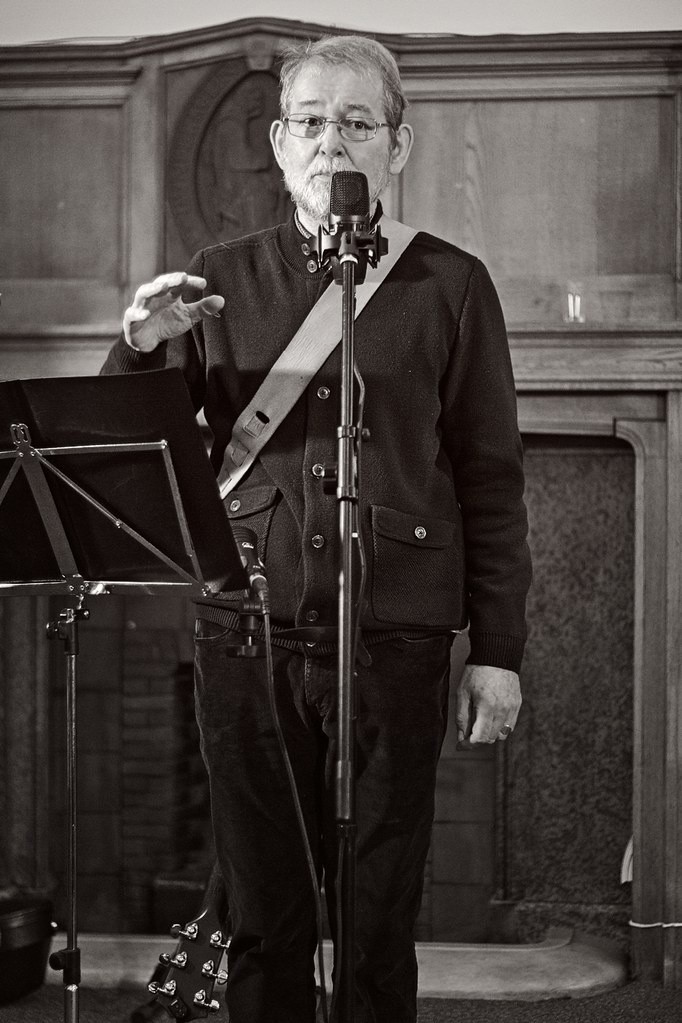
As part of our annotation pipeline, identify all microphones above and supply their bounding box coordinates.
[325,171,371,286]
[232,526,267,593]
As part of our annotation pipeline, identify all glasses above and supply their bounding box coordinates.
[281,113,396,141]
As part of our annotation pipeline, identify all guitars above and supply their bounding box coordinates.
[146,855,233,1023]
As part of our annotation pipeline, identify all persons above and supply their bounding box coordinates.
[97,35,535,1023]
